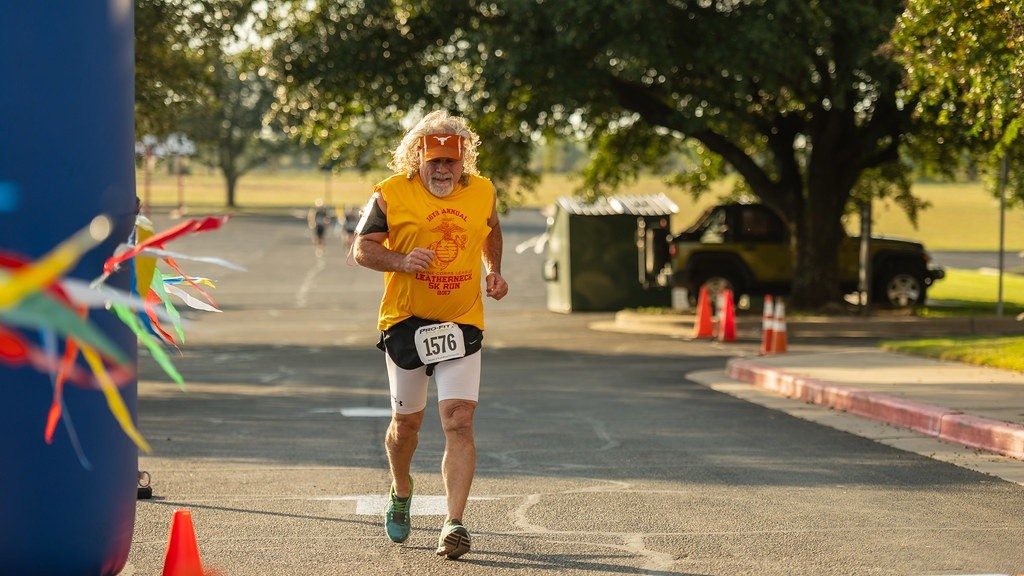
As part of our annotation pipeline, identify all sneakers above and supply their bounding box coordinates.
[436,519,471,559]
[384,474,414,542]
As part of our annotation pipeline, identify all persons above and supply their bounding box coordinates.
[354,108,510,560]
[307,199,362,249]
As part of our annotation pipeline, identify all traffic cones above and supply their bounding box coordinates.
[691,286,718,339]
[162,509,207,575]
[758,294,789,357]
[714,288,740,345]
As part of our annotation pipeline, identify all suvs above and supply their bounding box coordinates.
[669,203,944,315]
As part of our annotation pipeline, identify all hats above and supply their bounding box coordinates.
[418,134,465,161]
